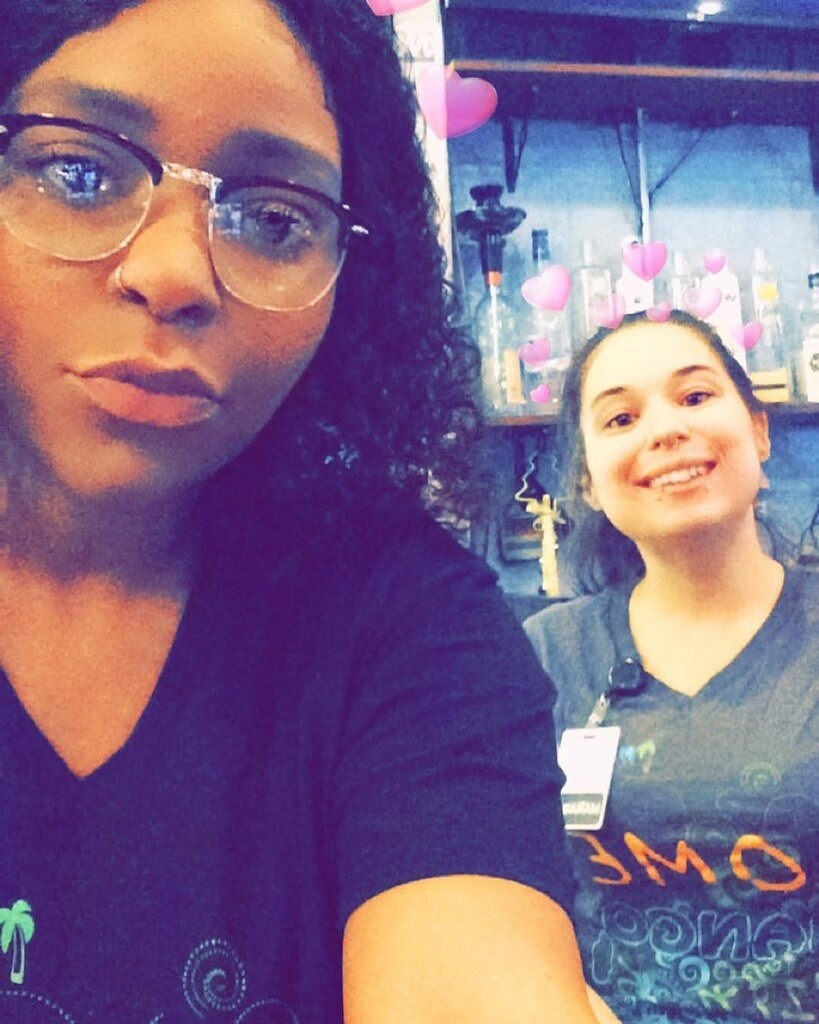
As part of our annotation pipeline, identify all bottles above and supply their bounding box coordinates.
[472,228,819,417]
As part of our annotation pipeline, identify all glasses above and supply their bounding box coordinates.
[0,114,369,313]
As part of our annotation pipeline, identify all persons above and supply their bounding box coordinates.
[520,308,819,1024]
[0,0,591,1024]
